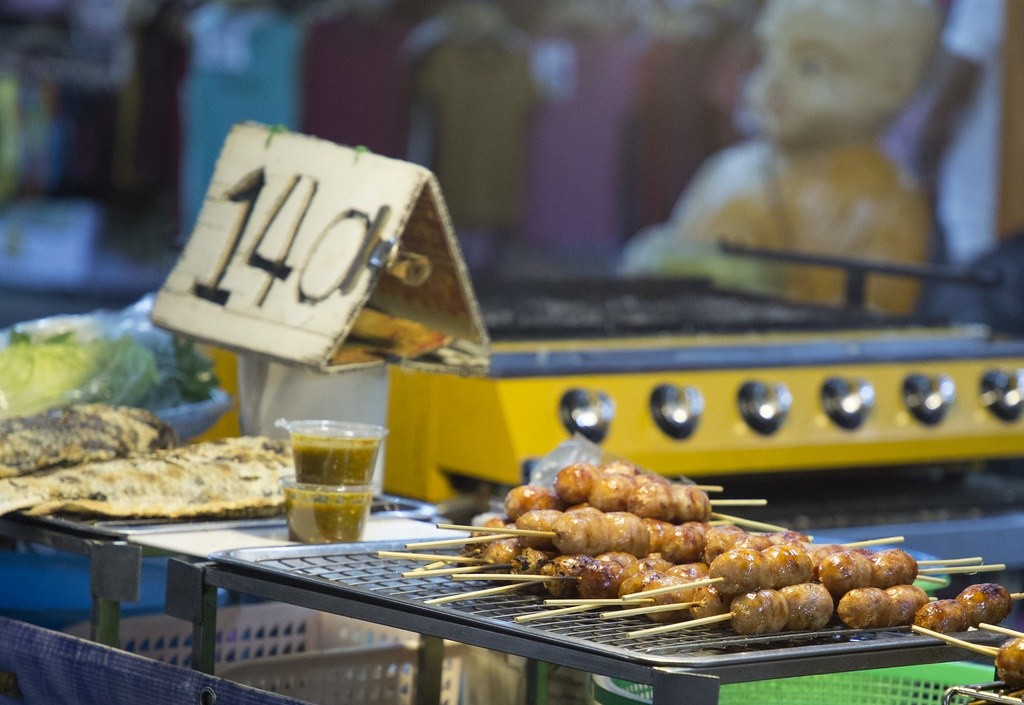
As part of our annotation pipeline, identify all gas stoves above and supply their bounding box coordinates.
[193,271,1024,501]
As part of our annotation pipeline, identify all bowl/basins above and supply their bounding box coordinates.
[278,476,378,543]
[274,417,391,486]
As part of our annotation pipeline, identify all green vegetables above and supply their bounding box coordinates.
[78,321,227,417]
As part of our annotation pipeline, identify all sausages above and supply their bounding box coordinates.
[452,461,1024,684]
